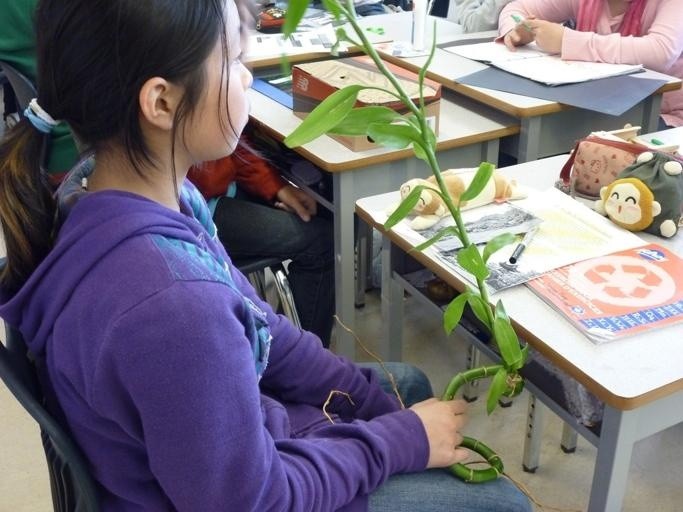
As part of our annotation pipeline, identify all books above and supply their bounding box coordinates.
[527,242,683,345]
[440,38,645,88]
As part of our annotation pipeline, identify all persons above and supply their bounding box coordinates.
[453,1,509,31]
[312,0,413,15]
[180,136,341,351]
[0,0,539,511]
[1,0,83,183]
[494,0,683,130]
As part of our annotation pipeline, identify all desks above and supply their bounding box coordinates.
[228,5,683,512]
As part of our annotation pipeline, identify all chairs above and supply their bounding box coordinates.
[0,237,305,512]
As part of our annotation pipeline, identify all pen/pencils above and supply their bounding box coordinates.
[650,137,663,147]
[511,14,532,33]
[441,303,487,342]
[509,226,540,264]
